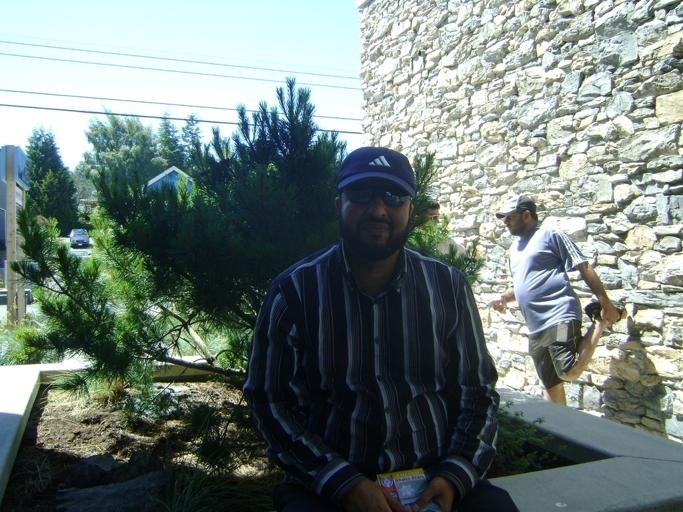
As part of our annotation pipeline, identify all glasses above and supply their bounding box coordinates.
[344,185,406,207]
[428,214,439,222]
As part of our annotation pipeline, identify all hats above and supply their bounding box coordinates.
[495,195,536,219]
[337,146,416,199]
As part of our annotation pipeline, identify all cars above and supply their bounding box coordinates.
[70,228,89,247]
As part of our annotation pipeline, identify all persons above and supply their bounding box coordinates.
[415,198,440,233]
[244,146,523,512]
[478,195,628,409]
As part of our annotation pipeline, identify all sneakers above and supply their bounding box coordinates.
[584,300,628,322]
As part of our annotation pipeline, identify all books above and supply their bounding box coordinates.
[374,466,442,512]
